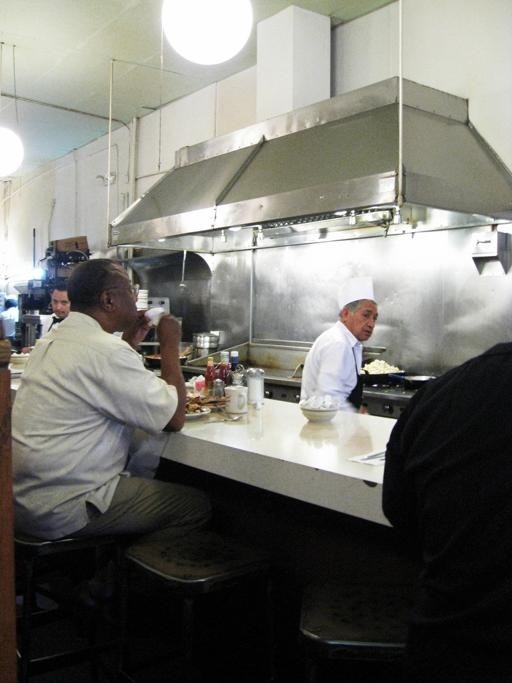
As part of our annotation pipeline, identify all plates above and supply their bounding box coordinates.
[185,406,212,422]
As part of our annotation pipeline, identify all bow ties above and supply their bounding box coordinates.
[52,317,64,323]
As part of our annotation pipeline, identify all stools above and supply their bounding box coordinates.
[13,534,127,683]
[121,533,276,683]
[297,533,406,683]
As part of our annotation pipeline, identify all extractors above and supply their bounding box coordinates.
[104,76,512,254]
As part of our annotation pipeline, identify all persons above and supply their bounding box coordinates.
[1,298,20,340]
[381,338,512,683]
[11,257,214,631]
[298,299,379,414]
[38,280,71,338]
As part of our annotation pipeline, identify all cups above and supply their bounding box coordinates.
[144,308,165,327]
[223,385,249,423]
[245,367,265,409]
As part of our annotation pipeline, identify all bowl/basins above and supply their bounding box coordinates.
[297,404,339,424]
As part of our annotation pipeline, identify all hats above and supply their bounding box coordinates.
[336,276,376,311]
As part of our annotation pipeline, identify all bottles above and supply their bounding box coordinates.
[204,350,241,397]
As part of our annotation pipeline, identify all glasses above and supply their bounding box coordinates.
[107,283,140,296]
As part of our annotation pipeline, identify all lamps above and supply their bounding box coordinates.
[162,0,252,67]
[0,41,24,178]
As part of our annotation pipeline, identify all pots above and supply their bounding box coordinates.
[142,353,188,369]
[192,332,219,349]
[356,370,438,388]
